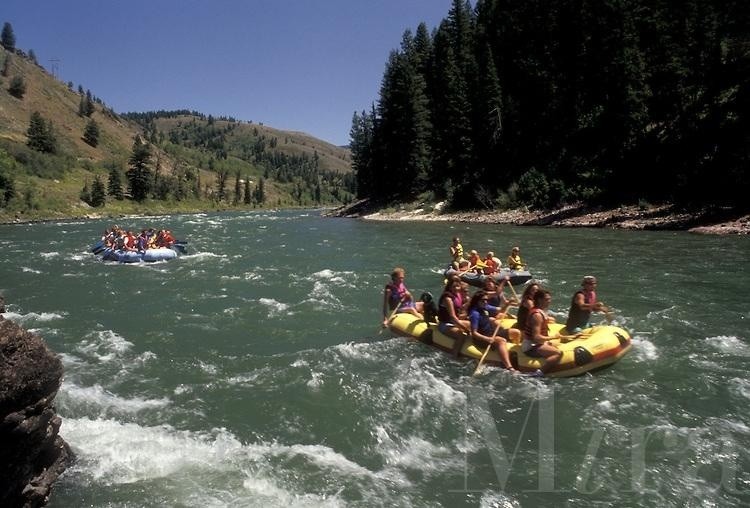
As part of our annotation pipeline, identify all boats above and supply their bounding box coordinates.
[443,264,533,286]
[387,310,634,379]
[103,244,179,262]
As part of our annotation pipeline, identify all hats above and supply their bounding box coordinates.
[581,276,594,286]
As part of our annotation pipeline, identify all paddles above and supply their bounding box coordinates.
[94,248,107,254]
[92,244,106,252]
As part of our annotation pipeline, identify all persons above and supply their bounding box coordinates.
[437,273,563,379]
[565,275,608,333]
[382,267,425,331]
[484,252,502,274]
[508,245,526,274]
[450,237,470,270]
[466,248,482,274]
[101,224,177,254]
